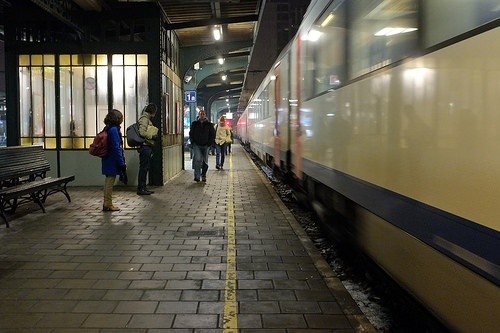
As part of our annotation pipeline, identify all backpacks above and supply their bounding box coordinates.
[89,125,116,157]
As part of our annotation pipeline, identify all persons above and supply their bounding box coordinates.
[209,123,233,155]
[102,109,127,210]
[137,104,157,195]
[214,116,231,170]
[190,110,216,182]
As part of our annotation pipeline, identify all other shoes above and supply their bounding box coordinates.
[137,189,151,195]
[144,188,155,193]
[103,204,120,211]
[216,165,220,169]
[202,178,206,182]
[221,165,223,169]
[194,178,200,182]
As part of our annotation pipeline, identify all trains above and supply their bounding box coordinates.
[236,0,500,333]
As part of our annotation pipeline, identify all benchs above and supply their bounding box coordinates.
[0,146,75,229]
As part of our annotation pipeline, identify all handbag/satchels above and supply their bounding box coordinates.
[126,115,149,147]
